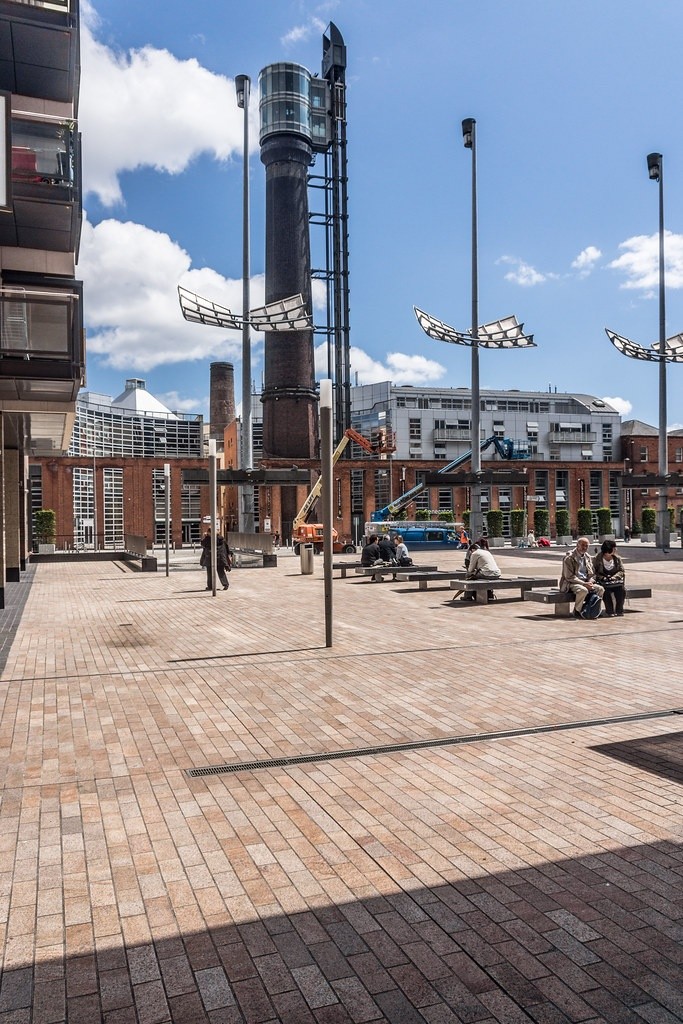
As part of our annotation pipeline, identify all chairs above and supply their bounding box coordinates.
[12,146,41,181]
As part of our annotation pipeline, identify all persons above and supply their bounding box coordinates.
[528,530,535,547]
[560,538,604,618]
[465,538,489,571]
[594,540,625,616]
[361,535,384,581]
[200,528,231,591]
[624,529,632,543]
[460,544,501,601]
[379,534,396,561]
[456,526,470,549]
[393,535,408,580]
[275,531,280,550]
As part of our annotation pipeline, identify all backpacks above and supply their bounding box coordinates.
[580,589,602,619]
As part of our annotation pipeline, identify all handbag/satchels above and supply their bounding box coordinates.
[398,557,412,567]
[227,553,232,564]
[605,579,623,587]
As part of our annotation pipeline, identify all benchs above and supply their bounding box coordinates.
[523,586,652,616]
[323,559,399,577]
[355,565,437,582]
[395,572,469,591]
[450,577,558,606]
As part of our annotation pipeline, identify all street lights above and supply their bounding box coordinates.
[605,154,683,549]
[413,117,539,545]
[624,458,630,525]
[178,75,313,534]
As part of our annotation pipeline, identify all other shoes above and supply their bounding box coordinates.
[460,597,472,601]
[205,587,212,590]
[609,613,615,617]
[616,612,624,616]
[223,584,229,590]
[371,579,376,582]
[573,608,581,618]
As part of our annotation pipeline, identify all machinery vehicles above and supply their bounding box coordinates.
[293,429,397,555]
[363,435,532,551]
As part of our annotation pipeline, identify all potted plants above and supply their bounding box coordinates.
[487,511,505,546]
[33,509,57,555]
[598,508,614,543]
[510,510,528,546]
[578,508,594,543]
[556,510,573,545]
[669,507,678,541]
[533,510,552,545]
[641,509,657,542]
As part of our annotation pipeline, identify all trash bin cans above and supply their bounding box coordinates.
[300,543,313,575]
